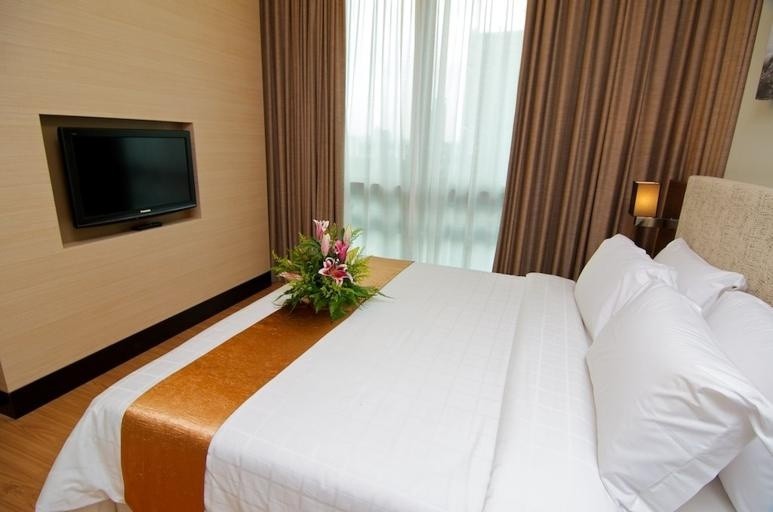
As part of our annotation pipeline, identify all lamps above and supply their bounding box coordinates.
[627,180,678,230]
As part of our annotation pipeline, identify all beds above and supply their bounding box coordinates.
[92,175,772,511]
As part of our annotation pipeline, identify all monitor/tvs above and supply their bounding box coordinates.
[54,126,196,230]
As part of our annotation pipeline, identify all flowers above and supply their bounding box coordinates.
[263,219,393,321]
